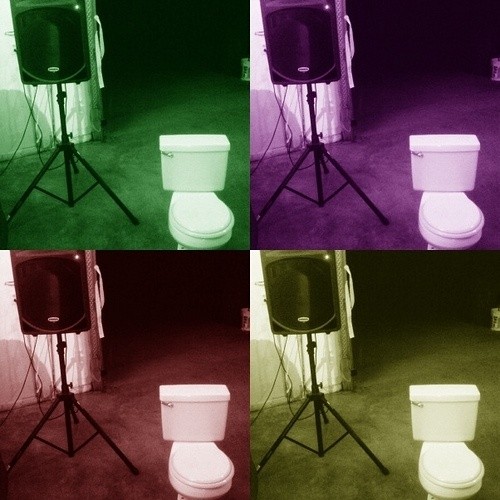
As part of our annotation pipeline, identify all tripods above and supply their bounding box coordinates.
[254,337,391,476]
[251,89,388,226]
[2,335,141,477]
[1,88,141,226]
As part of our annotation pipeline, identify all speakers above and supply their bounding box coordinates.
[9,0,92,87]
[9,251,92,338]
[259,0,342,88]
[259,249,341,335]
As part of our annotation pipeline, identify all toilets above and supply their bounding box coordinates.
[159,383,235,495]
[408,383,485,496]
[410,135,485,249]
